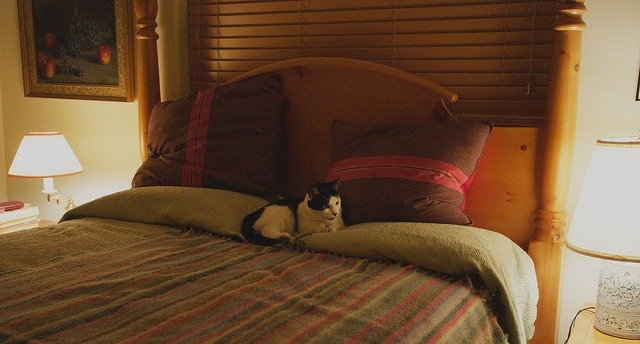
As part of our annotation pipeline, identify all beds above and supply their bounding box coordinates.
[1,0,589,343]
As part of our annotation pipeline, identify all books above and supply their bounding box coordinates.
[0,200,40,234]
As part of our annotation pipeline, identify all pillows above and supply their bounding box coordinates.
[322,117,493,224]
[131,69,285,197]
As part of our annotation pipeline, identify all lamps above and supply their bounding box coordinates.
[8,130,86,221]
[565,136,640,343]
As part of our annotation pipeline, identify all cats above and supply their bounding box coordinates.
[240,178,346,248]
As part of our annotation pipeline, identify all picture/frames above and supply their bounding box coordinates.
[16,0,136,101]
